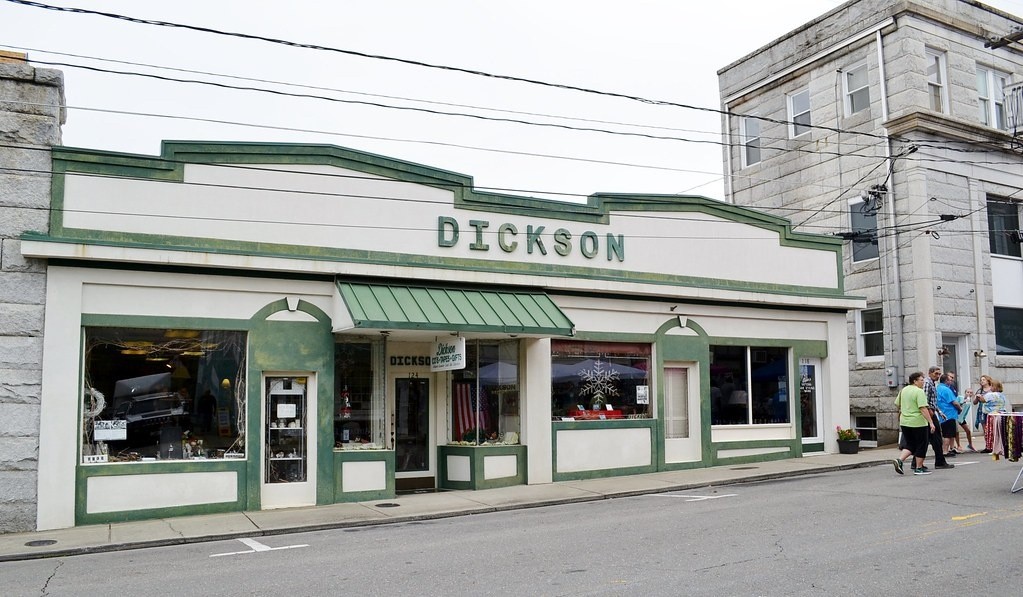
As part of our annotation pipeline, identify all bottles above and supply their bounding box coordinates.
[966,396,970,404]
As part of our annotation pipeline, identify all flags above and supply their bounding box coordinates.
[453,382,491,442]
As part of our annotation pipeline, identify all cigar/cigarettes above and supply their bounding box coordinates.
[946,419,948,420]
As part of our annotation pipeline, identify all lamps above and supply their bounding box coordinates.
[974,350,987,360]
[221,339,231,388]
[938,346,951,359]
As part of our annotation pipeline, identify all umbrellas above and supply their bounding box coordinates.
[480,362,517,386]
[554,359,648,383]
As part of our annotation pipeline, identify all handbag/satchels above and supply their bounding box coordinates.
[898,410,901,422]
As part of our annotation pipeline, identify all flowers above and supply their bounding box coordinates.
[836,425,860,440]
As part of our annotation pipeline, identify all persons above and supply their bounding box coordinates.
[956,388,978,452]
[178,387,189,398]
[978,378,1006,453]
[910,366,955,470]
[893,373,933,475]
[711,378,741,425]
[159,385,171,399]
[935,371,962,456]
[973,375,993,431]
[196,388,217,432]
[801,394,809,436]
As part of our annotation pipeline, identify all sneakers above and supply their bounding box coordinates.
[892,458,904,474]
[913,467,933,475]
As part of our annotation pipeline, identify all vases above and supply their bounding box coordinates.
[836,439,861,455]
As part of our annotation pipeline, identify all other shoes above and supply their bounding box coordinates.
[980,448,992,453]
[911,465,928,469]
[943,445,965,457]
[935,462,955,469]
[967,445,977,453]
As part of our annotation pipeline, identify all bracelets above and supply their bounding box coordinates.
[939,411,942,414]
